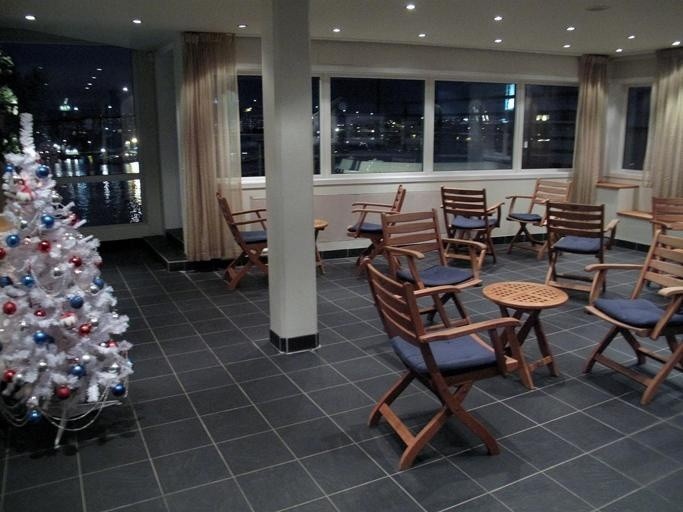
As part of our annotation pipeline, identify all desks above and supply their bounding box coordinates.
[480,281,568,389]
[312,219,327,274]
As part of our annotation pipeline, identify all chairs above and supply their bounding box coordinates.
[543,197,619,302]
[209,191,271,290]
[440,185,505,264]
[506,174,573,261]
[360,258,523,473]
[379,207,488,319]
[347,185,406,274]
[646,195,682,293]
[581,229,683,406]
